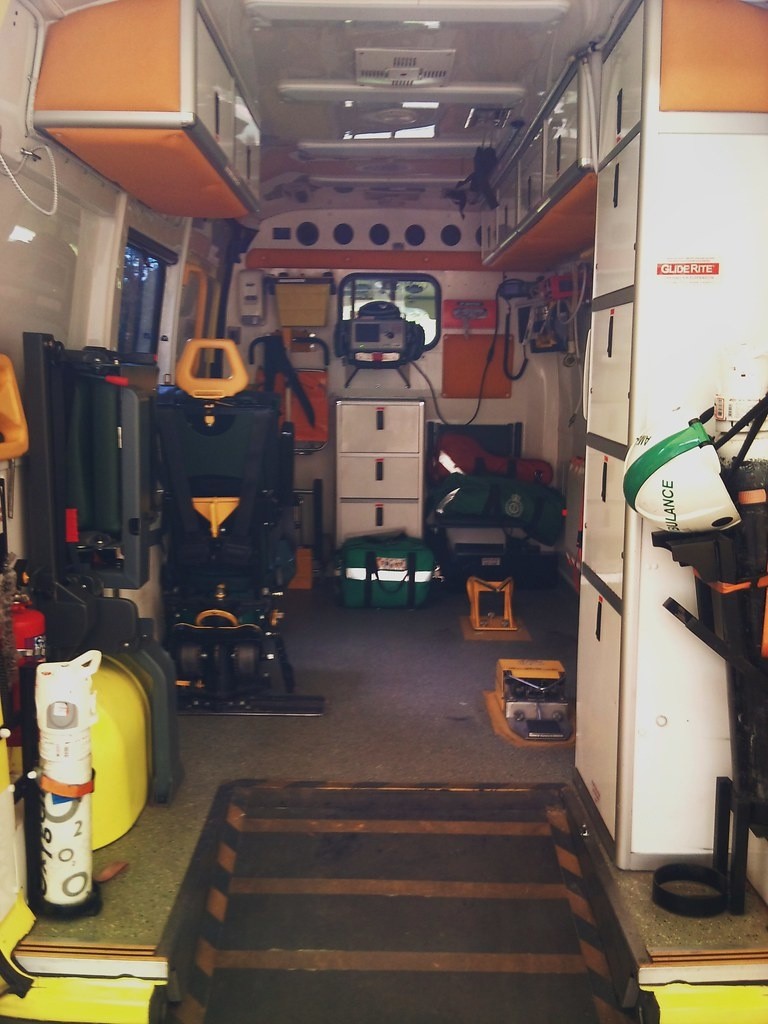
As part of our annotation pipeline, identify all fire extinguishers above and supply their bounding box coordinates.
[5,559,48,745]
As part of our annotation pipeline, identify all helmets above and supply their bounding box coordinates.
[623,403,742,535]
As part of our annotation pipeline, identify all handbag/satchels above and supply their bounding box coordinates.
[435,458,565,547]
[328,527,436,609]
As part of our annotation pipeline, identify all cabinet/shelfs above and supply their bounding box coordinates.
[475,59,599,274]
[566,2,767,877]
[26,0,263,221]
[334,398,423,543]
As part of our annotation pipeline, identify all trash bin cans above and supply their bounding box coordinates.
[652,465,767,835]
